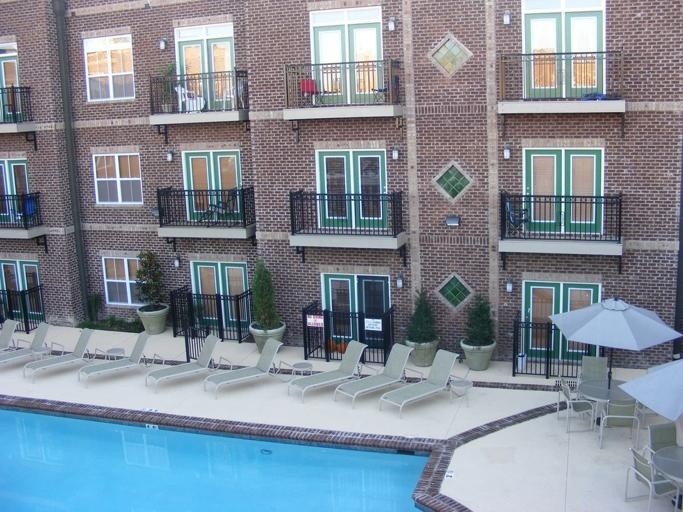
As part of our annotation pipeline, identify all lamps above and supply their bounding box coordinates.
[503,143,511,160]
[445,217,461,227]
[391,145,400,162]
[159,37,168,51]
[166,149,174,163]
[174,255,180,268]
[503,11,511,25]
[396,271,404,290]
[389,15,397,32]
[506,278,513,292]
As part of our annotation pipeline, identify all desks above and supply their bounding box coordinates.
[292,363,314,378]
[104,348,125,361]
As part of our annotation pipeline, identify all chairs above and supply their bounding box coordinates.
[203,337,285,396]
[558,357,683,512]
[23,327,93,381]
[0,321,49,368]
[288,341,368,405]
[503,193,528,239]
[334,342,414,406]
[0,318,19,353]
[379,346,461,419]
[145,334,222,395]
[9,193,37,227]
[77,331,153,384]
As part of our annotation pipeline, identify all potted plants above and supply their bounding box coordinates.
[138,251,170,333]
[459,294,497,372]
[249,263,286,352]
[405,287,440,368]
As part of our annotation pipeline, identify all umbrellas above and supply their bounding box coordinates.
[619,358,683,420]
[549,298,683,390]
